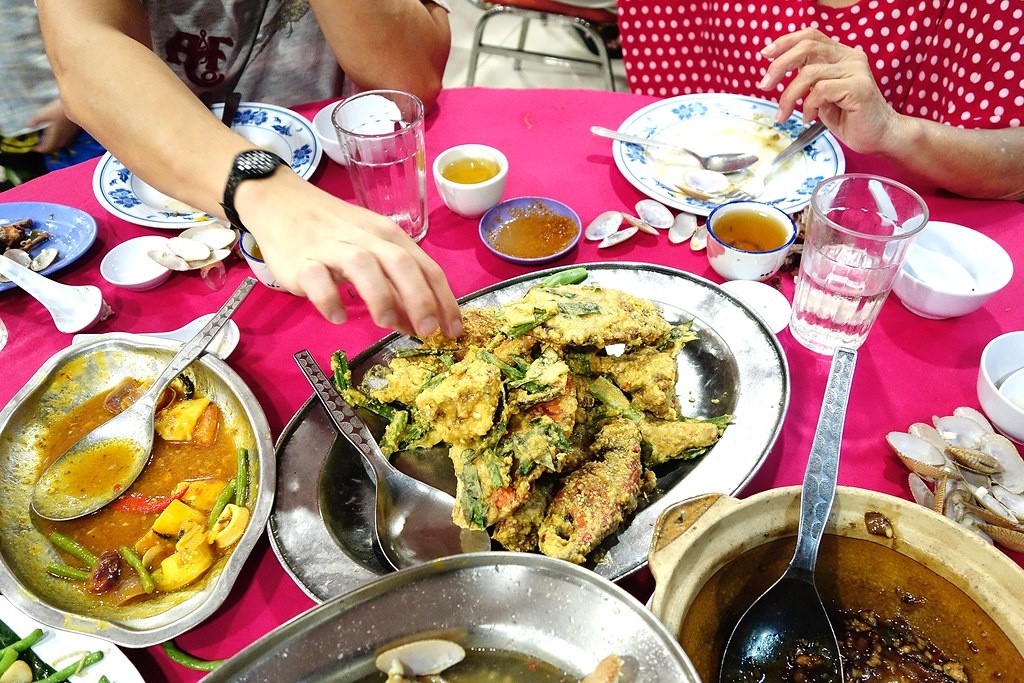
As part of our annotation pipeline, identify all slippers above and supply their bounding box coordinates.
[576,22,622,59]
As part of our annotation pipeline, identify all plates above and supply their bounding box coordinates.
[0,202,99,293]
[478,195,582,263]
[0,594,147,683]
[100,236,177,290]
[266,262,793,606]
[611,93,846,220]
[718,279,793,336]
[90,101,323,230]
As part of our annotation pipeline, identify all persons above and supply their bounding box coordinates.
[617,0,1024,204]
[0,0,106,189]
[556,0,622,59]
[36,0,462,343]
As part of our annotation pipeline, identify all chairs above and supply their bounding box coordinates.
[469,0,618,92]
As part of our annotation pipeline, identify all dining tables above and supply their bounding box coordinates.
[0,87,1024,683]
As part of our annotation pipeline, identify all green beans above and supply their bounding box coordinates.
[0,628,109,683]
[330,268,643,530]
[161,640,227,671]
[46,448,246,594]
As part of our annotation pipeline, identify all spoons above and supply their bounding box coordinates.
[71,312,240,362]
[998,366,1024,414]
[0,254,104,333]
[869,178,979,296]
[589,125,759,175]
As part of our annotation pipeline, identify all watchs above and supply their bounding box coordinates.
[221,150,293,234]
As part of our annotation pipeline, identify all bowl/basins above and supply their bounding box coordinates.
[0,332,277,648]
[643,481,1023,683]
[312,94,403,170]
[976,329,1024,446]
[193,552,706,683]
[883,220,1014,318]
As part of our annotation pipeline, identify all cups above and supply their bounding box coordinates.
[432,143,509,220]
[790,173,929,357]
[239,233,288,292]
[331,89,430,245]
[705,201,798,283]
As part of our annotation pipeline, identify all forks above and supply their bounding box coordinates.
[718,120,829,203]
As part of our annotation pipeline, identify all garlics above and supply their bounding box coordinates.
[0,660,32,683]
